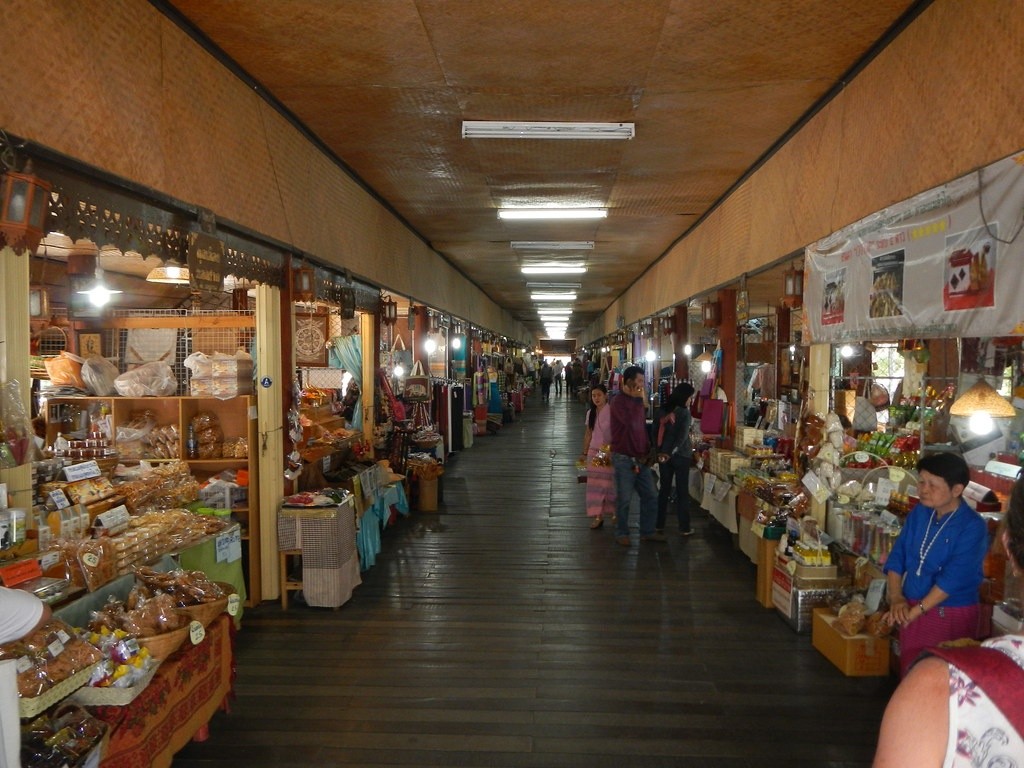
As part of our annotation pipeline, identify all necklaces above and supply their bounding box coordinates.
[916,506,957,575]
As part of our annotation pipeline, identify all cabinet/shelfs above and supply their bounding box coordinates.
[45,394,260,608]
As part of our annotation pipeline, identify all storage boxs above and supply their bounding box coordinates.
[758,534,891,676]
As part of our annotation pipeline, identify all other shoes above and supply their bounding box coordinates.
[617,537,633,547]
[640,533,669,541]
[611,514,618,524]
[680,529,695,536]
[589,520,603,528]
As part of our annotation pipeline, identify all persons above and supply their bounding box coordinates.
[564,358,589,393]
[883,453,987,679]
[506,359,513,382]
[579,384,618,528]
[31,327,68,414]
[611,366,668,543]
[871,466,1024,768]
[748,362,773,398]
[652,383,695,536]
[540,361,564,399]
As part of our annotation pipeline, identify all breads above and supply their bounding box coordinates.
[0,478,220,697]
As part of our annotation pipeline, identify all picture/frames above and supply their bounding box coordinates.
[293,312,329,368]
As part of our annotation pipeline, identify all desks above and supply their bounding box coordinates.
[53,523,242,651]
[86,612,234,768]
[651,464,791,568]
[277,499,354,612]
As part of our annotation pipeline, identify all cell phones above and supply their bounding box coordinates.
[626,380,641,392]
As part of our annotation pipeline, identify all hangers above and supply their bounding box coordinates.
[660,375,672,383]
[430,376,462,388]
[613,356,649,369]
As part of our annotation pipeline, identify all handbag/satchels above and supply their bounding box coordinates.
[389,335,414,370]
[404,360,432,401]
[504,357,514,375]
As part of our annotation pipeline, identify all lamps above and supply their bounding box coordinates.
[949,378,1015,435]
[463,120,635,141]
[496,209,609,221]
[380,295,529,350]
[74,251,124,307]
[702,297,718,329]
[512,241,594,338]
[145,259,189,284]
[0,126,50,256]
[781,259,803,308]
[695,349,714,372]
[290,258,315,302]
[762,304,774,342]
[591,312,674,349]
[334,286,356,320]
[29,271,51,333]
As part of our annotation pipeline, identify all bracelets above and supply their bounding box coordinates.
[919,600,927,615]
[583,452,587,456]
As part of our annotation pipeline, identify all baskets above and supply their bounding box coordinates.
[173,581,236,630]
[135,610,198,661]
